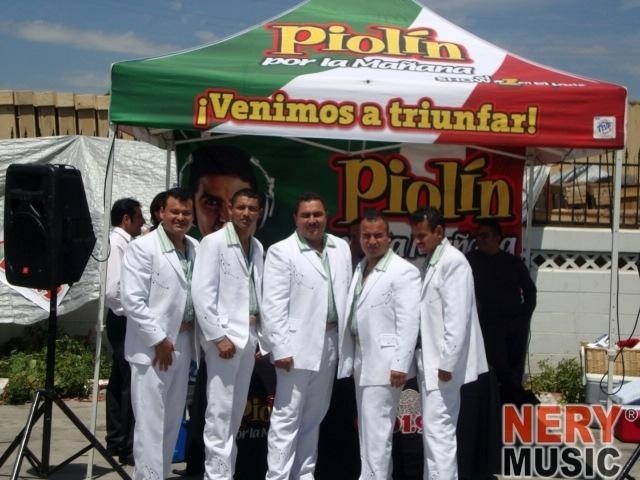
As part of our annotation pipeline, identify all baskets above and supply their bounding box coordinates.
[585,348,640,377]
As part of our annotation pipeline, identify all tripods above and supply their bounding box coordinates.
[0,288,132,480]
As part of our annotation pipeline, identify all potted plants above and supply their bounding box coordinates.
[523,353,585,405]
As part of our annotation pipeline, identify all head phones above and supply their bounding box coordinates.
[179,144,275,231]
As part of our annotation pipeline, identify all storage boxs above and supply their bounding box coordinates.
[584,372,640,429]
[613,403,640,443]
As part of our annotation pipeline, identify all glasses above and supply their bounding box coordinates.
[475,232,494,238]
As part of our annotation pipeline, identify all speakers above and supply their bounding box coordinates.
[4,163,97,290]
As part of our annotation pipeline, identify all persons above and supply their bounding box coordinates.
[106,189,488,479]
[194,145,256,241]
[465,218,541,403]
